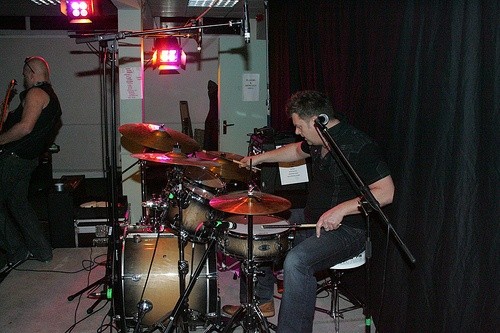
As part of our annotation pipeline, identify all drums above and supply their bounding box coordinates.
[112,231,219,328]
[218,216,292,262]
[159,166,225,243]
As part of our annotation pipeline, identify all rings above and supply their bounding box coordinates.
[325,227,328,229]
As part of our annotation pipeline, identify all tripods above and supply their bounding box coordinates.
[143,166,271,333]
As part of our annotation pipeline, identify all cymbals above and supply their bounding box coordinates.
[118,123,201,153]
[208,190,293,215]
[130,152,222,166]
[188,151,251,180]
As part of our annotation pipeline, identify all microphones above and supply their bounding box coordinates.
[202,220,236,229]
[315,114,329,125]
[243,0,251,44]
[197,18,203,53]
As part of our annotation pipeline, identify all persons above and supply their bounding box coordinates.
[223,91,394,333]
[0,57,63,261]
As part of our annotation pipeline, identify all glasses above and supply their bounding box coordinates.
[24,57,35,74]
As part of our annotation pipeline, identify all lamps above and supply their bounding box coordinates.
[151,37,187,70]
[61,0,95,23]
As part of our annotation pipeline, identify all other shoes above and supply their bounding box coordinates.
[222,300,274,318]
[29,254,40,260]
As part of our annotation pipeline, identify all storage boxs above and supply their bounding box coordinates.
[74,203,132,249]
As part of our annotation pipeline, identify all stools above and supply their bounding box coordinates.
[313,250,366,333]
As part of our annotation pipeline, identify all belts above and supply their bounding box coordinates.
[2,149,18,158]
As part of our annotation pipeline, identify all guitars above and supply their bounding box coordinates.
[0,79,17,130]
